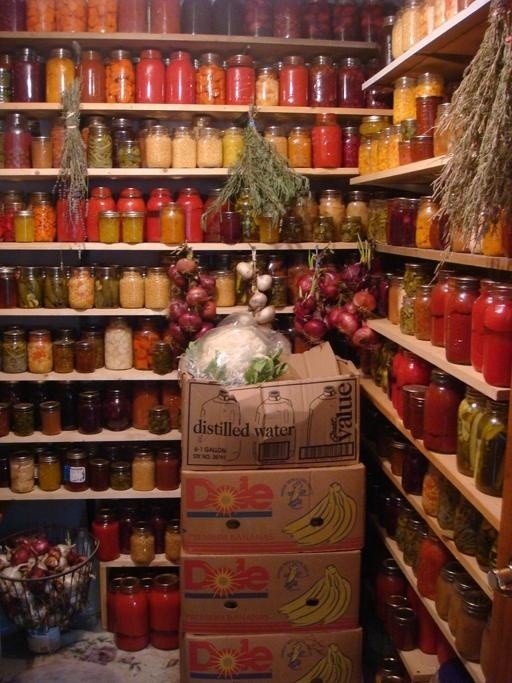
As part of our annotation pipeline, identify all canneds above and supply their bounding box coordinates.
[2,112,359,169]
[107,574,183,652]
[366,531,475,683]
[368,261,512,388]
[358,70,453,174]
[367,189,511,257]
[0,444,181,491]
[1,187,371,241]
[0,315,187,373]
[361,609,406,683]
[0,251,182,309]
[0,46,394,107]
[91,500,183,564]
[194,250,362,309]
[271,314,360,368]
[381,0,476,69]
[359,399,499,577]
[0,0,392,43]
[0,383,181,434]
[360,333,508,497]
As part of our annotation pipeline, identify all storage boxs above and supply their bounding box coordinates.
[177,463,369,557]
[175,546,367,636]
[180,632,366,680]
[172,344,367,474]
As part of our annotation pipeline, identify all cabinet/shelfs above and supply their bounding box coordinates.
[1,0,387,632]
[346,0,510,683]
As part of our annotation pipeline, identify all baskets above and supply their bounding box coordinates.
[0,525,101,634]
